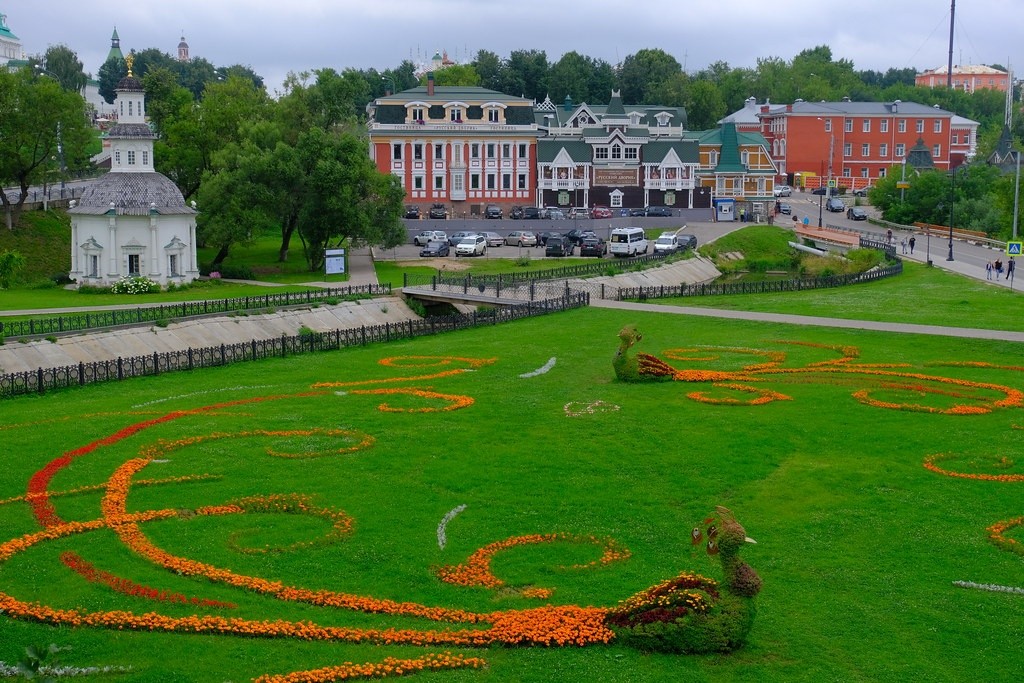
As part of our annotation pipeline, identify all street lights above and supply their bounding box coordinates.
[818,117,830,198]
[35,64,66,199]
[902,155,906,202]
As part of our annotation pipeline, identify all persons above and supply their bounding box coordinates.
[740,208,747,222]
[902,235,908,254]
[909,235,915,254]
[777,200,781,212]
[887,229,892,238]
[536,232,543,248]
[995,258,1002,280]
[1006,257,1015,280]
[986,261,994,280]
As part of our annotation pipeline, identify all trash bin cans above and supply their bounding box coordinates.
[928,261,933,266]
[620,209,626,217]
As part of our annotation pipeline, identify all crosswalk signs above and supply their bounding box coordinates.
[1007,242,1022,256]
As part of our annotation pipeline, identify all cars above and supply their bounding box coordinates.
[485,204,503,219]
[420,241,449,257]
[429,203,447,219]
[774,186,792,214]
[535,229,607,258]
[503,231,537,247]
[477,232,504,247]
[455,236,486,257]
[653,234,697,253]
[414,231,448,246]
[509,205,612,219]
[620,205,672,217]
[448,231,476,246]
[402,204,420,218]
[811,185,873,221]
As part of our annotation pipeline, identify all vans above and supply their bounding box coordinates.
[609,227,648,257]
